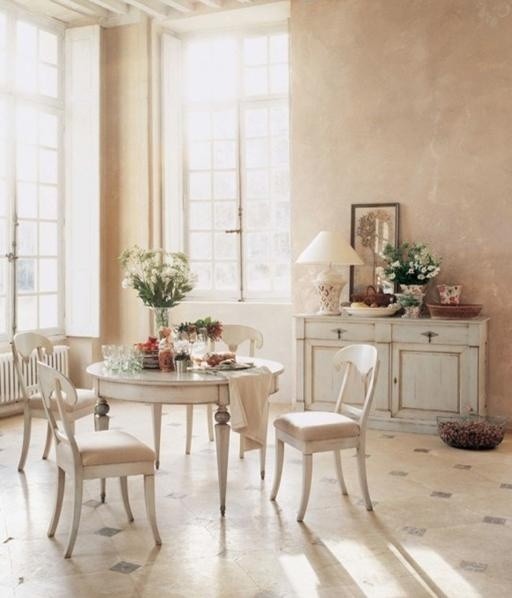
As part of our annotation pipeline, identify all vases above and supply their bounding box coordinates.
[175,317,223,342]
[380,240,442,285]
[117,243,197,326]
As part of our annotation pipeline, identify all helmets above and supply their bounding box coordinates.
[343,306,399,318]
[428,303,479,318]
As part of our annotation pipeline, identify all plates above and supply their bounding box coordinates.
[291,314,490,436]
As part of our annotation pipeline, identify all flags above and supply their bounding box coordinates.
[400,285,427,319]
[437,284,463,304]
[186,324,263,459]
[146,306,171,338]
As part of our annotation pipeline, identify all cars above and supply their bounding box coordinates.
[176,360,187,372]
[100,343,144,376]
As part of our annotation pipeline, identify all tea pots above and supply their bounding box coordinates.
[350,202,399,303]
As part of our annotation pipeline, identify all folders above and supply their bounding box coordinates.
[0,345,71,404]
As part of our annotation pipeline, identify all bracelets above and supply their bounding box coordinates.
[140,354,159,369]
[436,416,509,449]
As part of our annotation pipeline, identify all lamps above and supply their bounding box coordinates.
[269,344,380,522]
[37,361,161,559]
[10,332,97,473]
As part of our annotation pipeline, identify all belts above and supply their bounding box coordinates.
[176,339,208,369]
